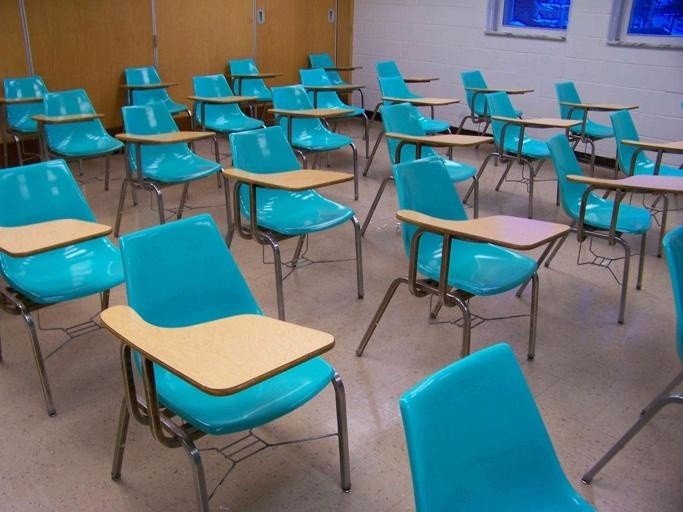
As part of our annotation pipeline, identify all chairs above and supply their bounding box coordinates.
[298,67,370,159]
[604,110,683,203]
[308,52,364,109]
[227,57,272,118]
[463,91,562,218]
[0,76,49,165]
[359,102,480,237]
[110,214,351,512]
[42,88,124,192]
[190,74,266,133]
[353,155,542,359]
[0,160,124,415]
[114,101,233,239]
[223,124,369,319]
[583,223,683,482]
[534,82,615,177]
[125,66,192,150]
[362,75,454,177]
[398,344,601,511]
[363,60,434,140]
[448,71,521,155]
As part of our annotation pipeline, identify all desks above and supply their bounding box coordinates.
[306,85,365,102]
[115,132,219,191]
[323,66,359,73]
[464,87,533,122]
[233,73,283,95]
[187,95,261,132]
[94,306,335,448]
[119,83,178,107]
[396,209,571,306]
[494,117,585,166]
[222,166,352,245]
[0,218,112,257]
[387,132,495,165]
[268,108,355,144]
[569,176,683,245]
[623,139,683,175]
[31,113,106,158]
[561,99,639,143]
[384,96,459,107]
[402,76,439,84]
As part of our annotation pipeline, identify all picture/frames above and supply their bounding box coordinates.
[484,0,572,42]
[605,0,683,51]
